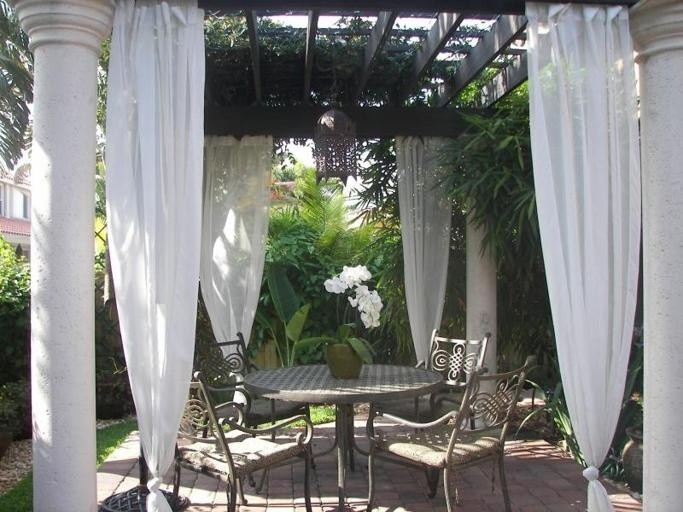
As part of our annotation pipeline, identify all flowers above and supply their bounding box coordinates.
[294,265,384,365]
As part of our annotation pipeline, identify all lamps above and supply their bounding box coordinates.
[313,81,360,185]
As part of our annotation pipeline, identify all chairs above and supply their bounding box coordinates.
[169,371,313,512]
[349,328,491,499]
[196,333,315,487]
[365,356,534,512]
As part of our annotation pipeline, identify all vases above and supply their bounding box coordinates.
[326,344,362,379]
[622,426,643,493]
